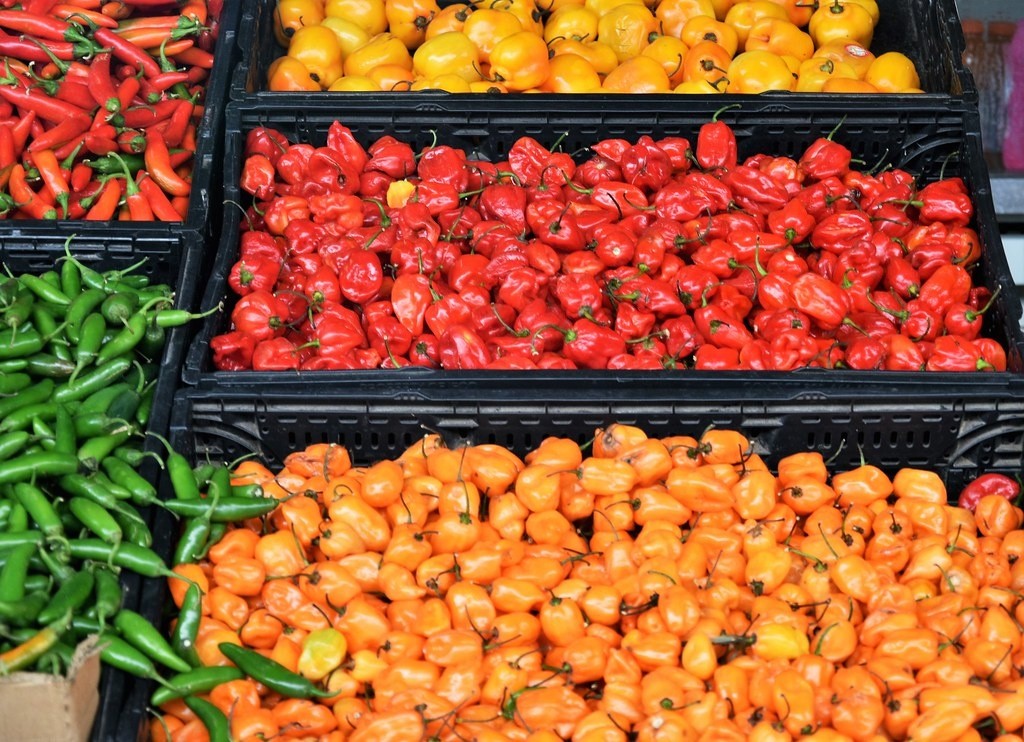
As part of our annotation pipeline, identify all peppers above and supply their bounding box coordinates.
[0,2,1023,742]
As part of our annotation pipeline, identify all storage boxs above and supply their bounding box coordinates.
[0,0,1023,741]
